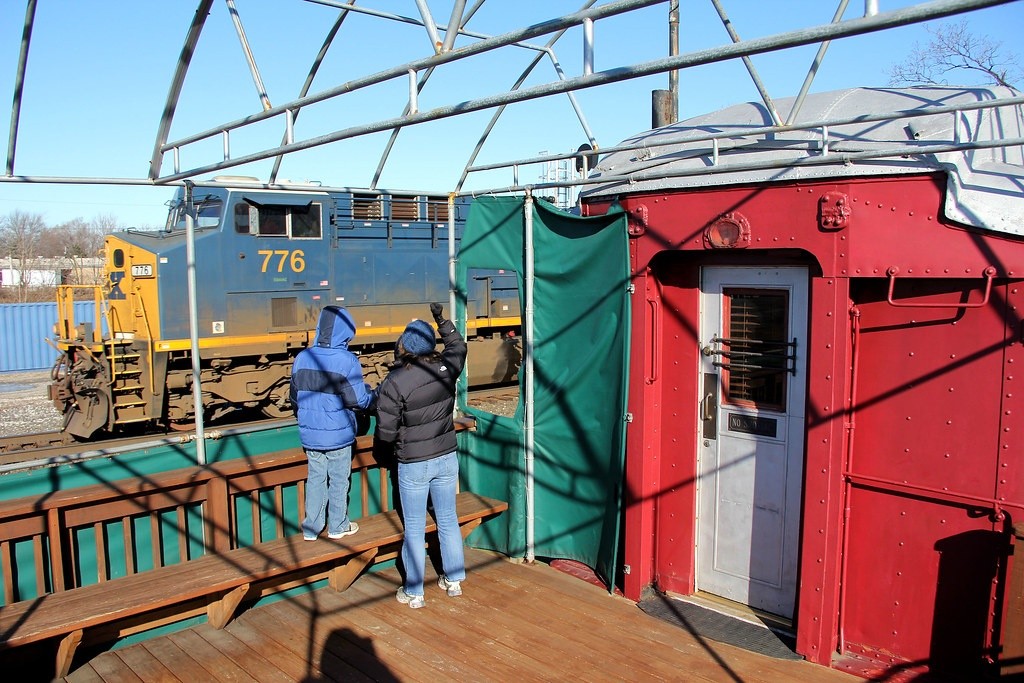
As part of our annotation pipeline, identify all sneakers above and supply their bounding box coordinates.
[437,575,462,596]
[395,586,426,608]
[328,522,359,539]
[304,536,317,541]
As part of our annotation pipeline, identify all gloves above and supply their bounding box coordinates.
[430,302,445,327]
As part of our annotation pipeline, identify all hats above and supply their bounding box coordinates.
[403,318,436,354]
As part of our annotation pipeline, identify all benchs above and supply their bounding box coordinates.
[0,417,508,679]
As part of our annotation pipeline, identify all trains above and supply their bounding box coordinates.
[45,176,580,442]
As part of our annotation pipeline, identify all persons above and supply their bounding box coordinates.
[375,301,467,608]
[289,305,373,541]
